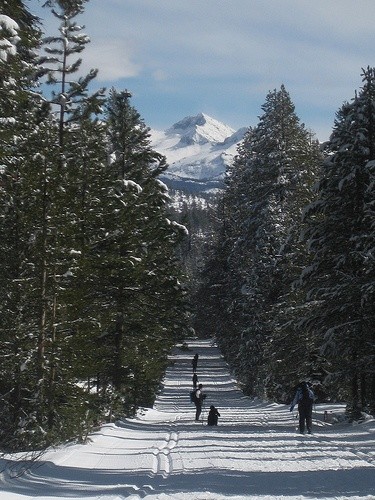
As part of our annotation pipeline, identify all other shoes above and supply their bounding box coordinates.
[299,431,304,434]
[307,430,313,434]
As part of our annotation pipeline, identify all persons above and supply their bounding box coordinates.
[208,405,221,426]
[192,356,197,372]
[289,382,316,435]
[192,374,198,388]
[195,353,198,361]
[191,384,206,423]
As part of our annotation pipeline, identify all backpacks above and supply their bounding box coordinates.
[191,391,196,403]
